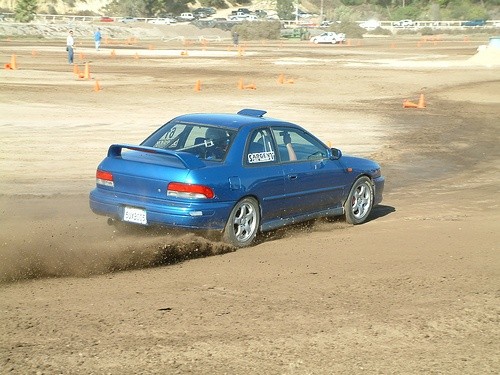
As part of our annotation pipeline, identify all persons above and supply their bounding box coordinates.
[95,28,102,50]
[67,30,76,63]
[232,31,240,47]
[258,132,298,162]
[203,127,227,160]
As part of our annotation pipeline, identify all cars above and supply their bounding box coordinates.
[87,106,385,249]
[310,32,347,44]
[75,7,344,27]
[356,15,492,33]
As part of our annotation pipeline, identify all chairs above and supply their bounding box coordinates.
[191,134,269,163]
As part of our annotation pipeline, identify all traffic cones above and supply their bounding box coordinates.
[245,83,257,89]
[238,78,243,89]
[288,78,294,83]
[194,80,201,90]
[402,100,417,109]
[96,81,100,91]
[280,73,284,84]
[417,93,426,109]
[3,63,10,69]
[11,52,16,69]
[74,64,91,81]
